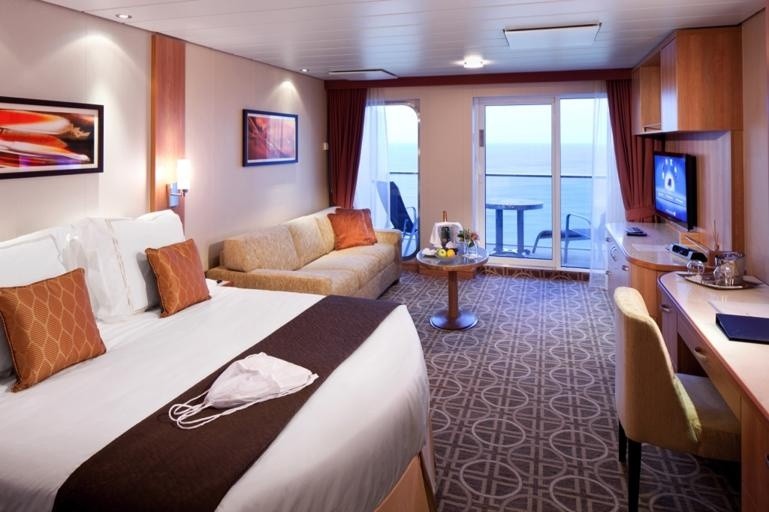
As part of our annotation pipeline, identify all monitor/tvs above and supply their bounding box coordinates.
[652,150,698,233]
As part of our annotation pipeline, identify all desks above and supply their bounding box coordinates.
[657,271,769,512]
[486,198,544,257]
[416,246,489,332]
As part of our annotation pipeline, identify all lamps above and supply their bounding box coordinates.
[464,61,484,68]
[166,159,192,208]
[503,23,602,49]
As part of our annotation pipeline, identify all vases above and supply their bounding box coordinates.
[464,241,478,258]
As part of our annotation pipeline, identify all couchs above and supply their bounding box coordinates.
[206,207,402,300]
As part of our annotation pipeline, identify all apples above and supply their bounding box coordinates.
[447,249,455,257]
[438,249,447,257]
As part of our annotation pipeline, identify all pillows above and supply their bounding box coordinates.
[223,206,377,279]
[0,209,211,392]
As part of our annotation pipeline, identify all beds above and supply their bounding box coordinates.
[0,217,436,512]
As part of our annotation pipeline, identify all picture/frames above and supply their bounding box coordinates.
[243,109,298,166]
[0,96,103,179]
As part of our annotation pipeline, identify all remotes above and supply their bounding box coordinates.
[627,232,647,236]
[632,226,641,233]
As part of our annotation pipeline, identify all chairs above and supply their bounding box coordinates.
[533,212,606,270]
[613,287,740,512]
[372,180,418,261]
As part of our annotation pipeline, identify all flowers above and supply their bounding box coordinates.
[457,227,480,247]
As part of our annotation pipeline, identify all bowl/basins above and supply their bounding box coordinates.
[434,254,457,262]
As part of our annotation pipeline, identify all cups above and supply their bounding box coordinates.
[714,265,733,287]
[687,260,705,279]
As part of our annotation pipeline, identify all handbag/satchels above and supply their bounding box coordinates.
[168,351,320,431]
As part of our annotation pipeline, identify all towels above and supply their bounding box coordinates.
[430,222,463,249]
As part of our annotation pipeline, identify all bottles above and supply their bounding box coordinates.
[439,210,450,248]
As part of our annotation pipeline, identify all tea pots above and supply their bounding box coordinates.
[715,253,744,285]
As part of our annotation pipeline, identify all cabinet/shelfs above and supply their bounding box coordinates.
[632,24,743,135]
[606,222,716,329]
